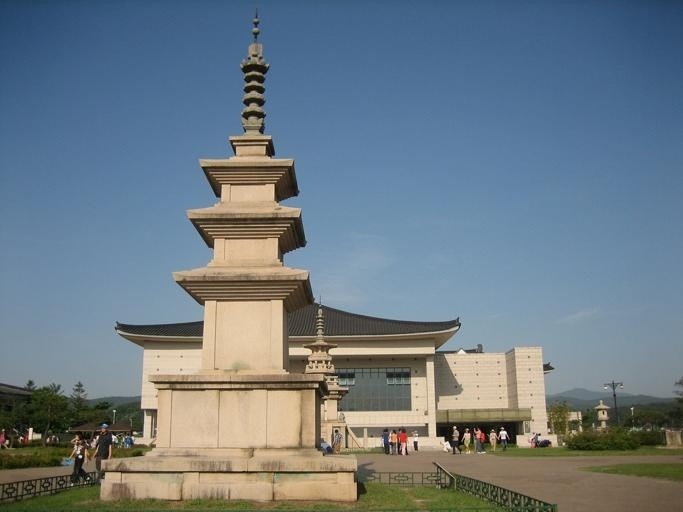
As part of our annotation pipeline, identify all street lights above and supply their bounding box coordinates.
[128,417,133,428]
[602,379,625,425]
[629,405,635,429]
[111,408,117,424]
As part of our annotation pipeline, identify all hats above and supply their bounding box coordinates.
[500,427,504,429]
[100,424,108,429]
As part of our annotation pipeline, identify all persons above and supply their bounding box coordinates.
[527,432,552,449]
[460,427,510,454]
[69,423,134,488]
[411,430,419,453]
[451,425,461,455]
[333,429,344,455]
[0,428,8,449]
[383,427,410,455]
